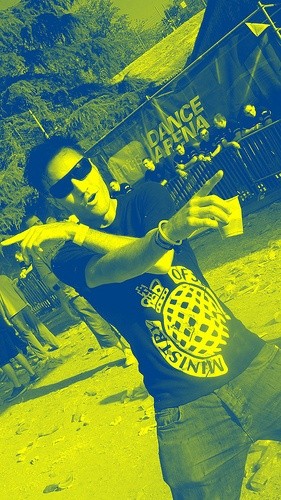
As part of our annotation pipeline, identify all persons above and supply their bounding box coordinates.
[242,104,272,134]
[0,210,138,402]
[109,179,135,199]
[212,113,245,149]
[0,134,280,500]
[140,157,172,185]
[198,126,222,161]
[172,141,198,179]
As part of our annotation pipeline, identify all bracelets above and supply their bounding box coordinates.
[157,220,183,246]
[73,223,89,246]
[155,229,173,250]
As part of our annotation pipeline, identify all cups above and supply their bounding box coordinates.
[214,195,244,239]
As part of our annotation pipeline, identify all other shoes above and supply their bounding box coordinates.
[48,342,62,352]
[36,354,48,365]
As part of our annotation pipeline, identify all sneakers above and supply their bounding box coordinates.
[7,384,26,403]
[124,348,136,366]
[29,371,40,384]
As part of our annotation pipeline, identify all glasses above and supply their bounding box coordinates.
[45,153,91,199]
